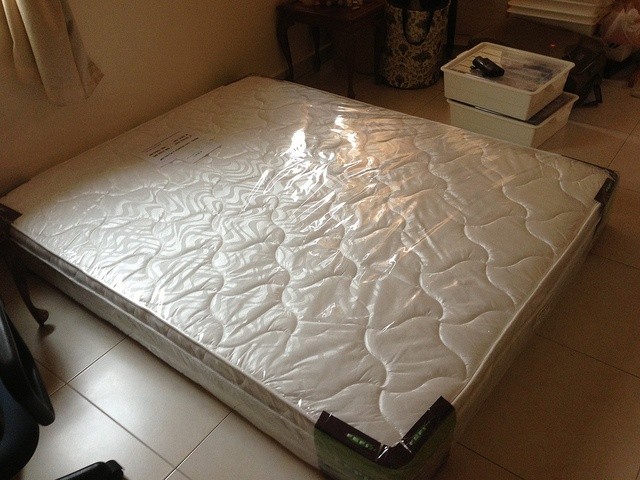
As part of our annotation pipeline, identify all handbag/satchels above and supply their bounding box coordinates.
[562,36,606,108]
[376,1,450,90]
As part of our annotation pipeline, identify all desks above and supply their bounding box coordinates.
[275,2,385,99]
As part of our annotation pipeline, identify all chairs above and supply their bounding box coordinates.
[0,306,124,480]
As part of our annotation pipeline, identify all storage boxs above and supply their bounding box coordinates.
[446,90,580,150]
[440,41,576,122]
